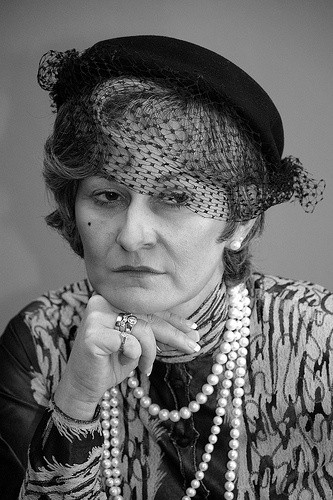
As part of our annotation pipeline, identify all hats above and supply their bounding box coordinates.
[34,35,326,221]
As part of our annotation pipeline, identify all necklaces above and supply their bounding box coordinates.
[100,274,251,500]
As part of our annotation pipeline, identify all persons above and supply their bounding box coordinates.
[0,35,332,500]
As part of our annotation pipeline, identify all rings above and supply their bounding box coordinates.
[115,312,138,333]
[118,332,127,354]
